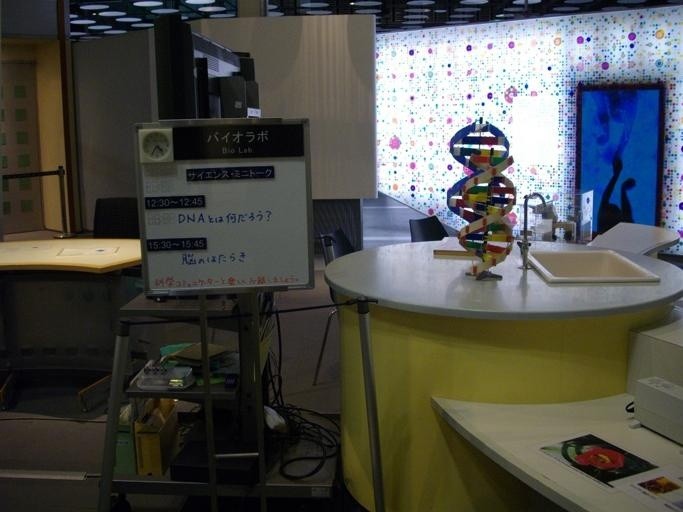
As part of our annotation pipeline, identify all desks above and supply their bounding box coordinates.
[318,218,682,511]
[0,231,162,409]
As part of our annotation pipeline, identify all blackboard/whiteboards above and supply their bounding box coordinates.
[132,117,315,298]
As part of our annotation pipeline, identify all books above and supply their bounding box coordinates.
[174,342,228,366]
[433,236,475,257]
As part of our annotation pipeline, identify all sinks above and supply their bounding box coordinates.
[529,249,661,285]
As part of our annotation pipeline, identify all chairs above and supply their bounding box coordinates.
[407,215,450,242]
[311,225,356,390]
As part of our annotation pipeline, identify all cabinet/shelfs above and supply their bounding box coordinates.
[77,279,353,509]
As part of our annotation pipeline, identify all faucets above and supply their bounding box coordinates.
[517,193,546,268]
